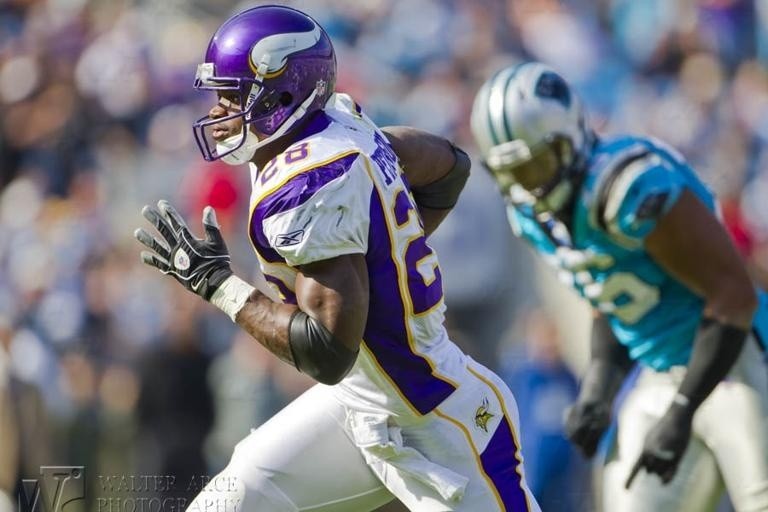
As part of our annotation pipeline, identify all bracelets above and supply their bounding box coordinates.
[206,271,257,326]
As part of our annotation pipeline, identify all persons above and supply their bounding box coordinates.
[505,310,599,510]
[470,62,767,511]
[134,295,217,510]
[132,5,543,509]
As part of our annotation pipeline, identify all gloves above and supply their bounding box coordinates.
[133,198,256,321]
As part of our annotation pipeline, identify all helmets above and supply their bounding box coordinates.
[467,60,593,200]
[202,4,337,138]
